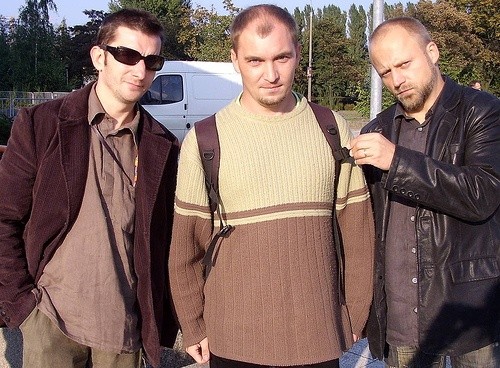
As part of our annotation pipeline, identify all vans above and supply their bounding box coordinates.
[138,61,243,148]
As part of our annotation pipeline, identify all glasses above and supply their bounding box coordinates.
[100,44,165,71]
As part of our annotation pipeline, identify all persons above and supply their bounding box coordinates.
[349,18,500,368]
[168,5,375,368]
[0,9,179,368]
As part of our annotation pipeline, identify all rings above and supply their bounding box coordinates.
[364,149,367,158]
